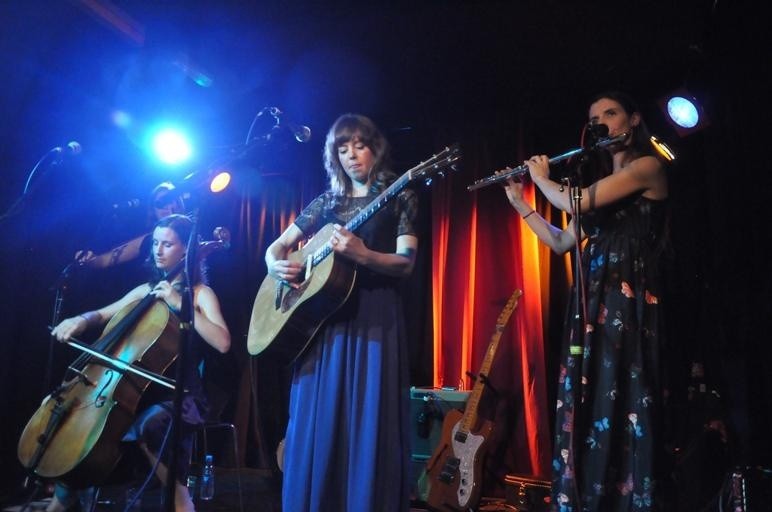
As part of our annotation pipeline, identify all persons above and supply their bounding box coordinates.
[73,182,185,269]
[264,111,422,512]
[493,90,729,512]
[42,212,232,512]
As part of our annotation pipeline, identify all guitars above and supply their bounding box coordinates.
[246,147,471,373]
[418,291,524,510]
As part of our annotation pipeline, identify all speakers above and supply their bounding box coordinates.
[409,387,472,460]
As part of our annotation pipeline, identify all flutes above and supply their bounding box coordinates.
[467,131,628,193]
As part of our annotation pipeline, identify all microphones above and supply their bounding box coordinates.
[112,198,139,209]
[269,105,312,144]
[417,404,432,439]
[62,140,82,156]
[589,123,609,138]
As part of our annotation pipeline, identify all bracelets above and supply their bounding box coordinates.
[79,310,103,327]
[523,210,534,219]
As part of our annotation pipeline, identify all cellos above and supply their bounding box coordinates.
[19,225,232,488]
